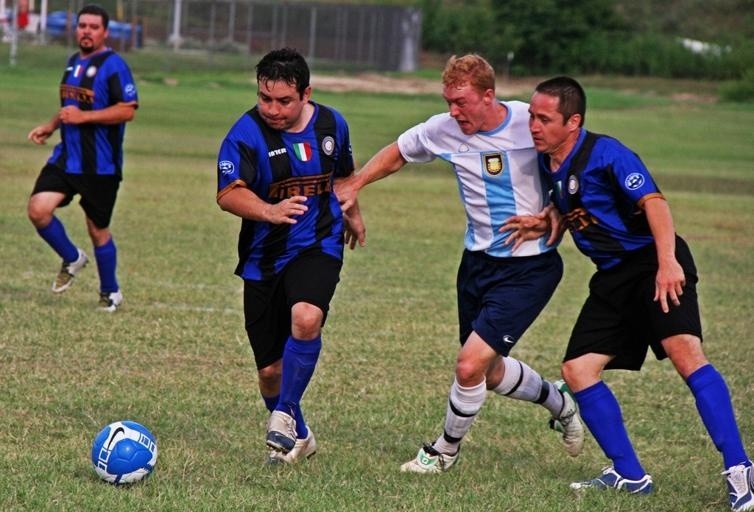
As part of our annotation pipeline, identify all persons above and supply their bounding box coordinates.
[216,48,367,465]
[334,53,584,475]
[499,76,752,511]
[26,5,140,313]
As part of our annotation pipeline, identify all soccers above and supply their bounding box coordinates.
[90,421,157,484]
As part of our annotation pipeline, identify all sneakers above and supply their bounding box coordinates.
[95,289,123,312]
[269,424,316,465]
[569,466,653,496]
[400,441,461,474]
[548,379,586,457]
[265,409,298,455]
[720,460,754,512]
[52,248,89,295]
